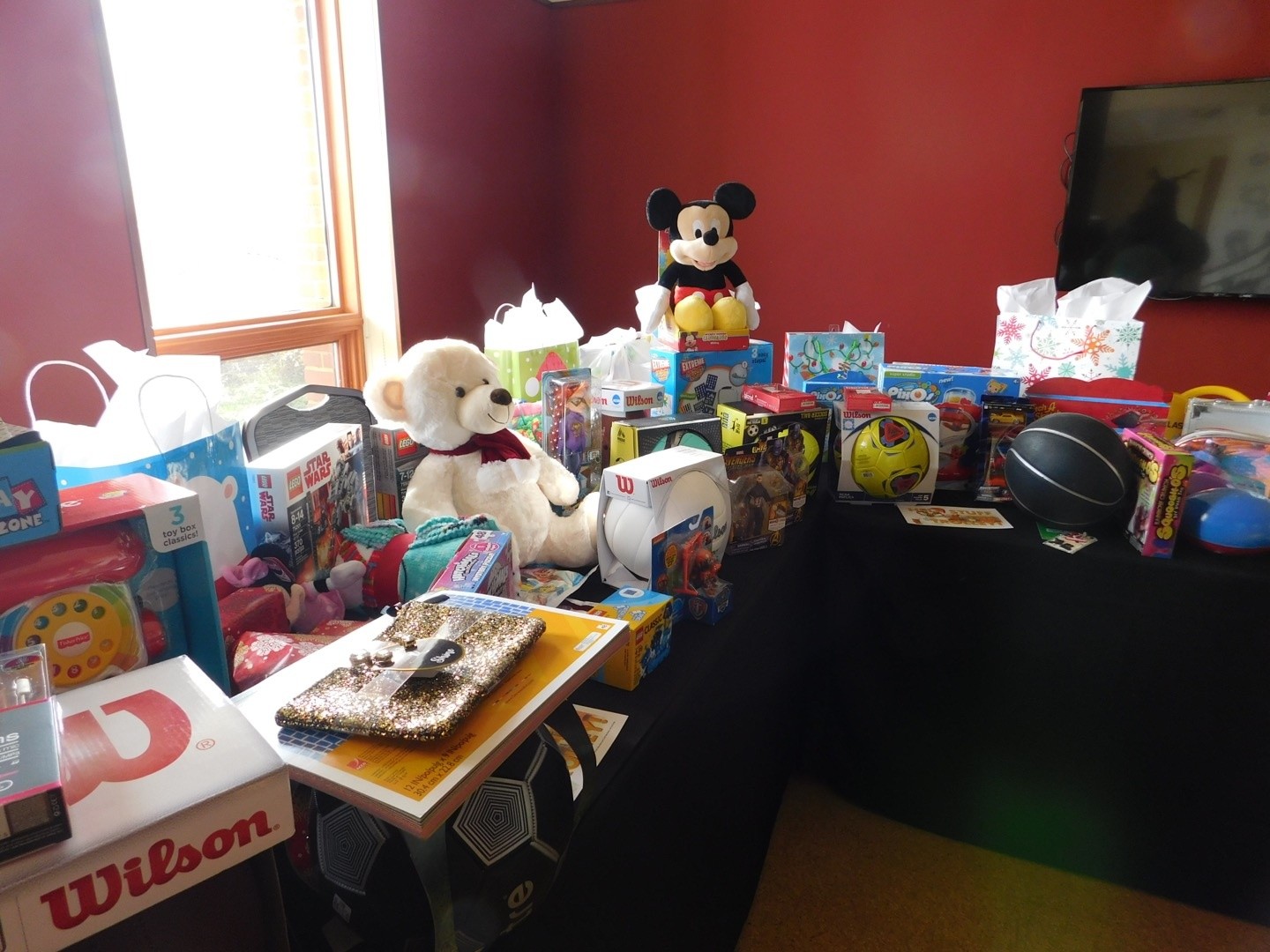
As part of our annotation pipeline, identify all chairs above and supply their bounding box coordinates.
[244,384,389,523]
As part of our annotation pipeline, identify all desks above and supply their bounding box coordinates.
[523,518,823,886]
[833,502,1270,775]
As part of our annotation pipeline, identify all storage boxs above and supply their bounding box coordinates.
[607,413,722,470]
[660,336,774,412]
[243,423,368,581]
[1120,426,1193,560]
[0,475,233,690]
[600,378,665,468]
[975,393,1026,500]
[372,422,432,519]
[717,398,832,514]
[885,359,1020,494]
[0,655,297,952]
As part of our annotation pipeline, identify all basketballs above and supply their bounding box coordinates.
[1005,412,1133,534]
[607,474,729,582]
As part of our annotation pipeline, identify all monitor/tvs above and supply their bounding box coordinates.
[1056,75,1269,300]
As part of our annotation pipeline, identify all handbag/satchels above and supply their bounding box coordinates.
[781,329,885,390]
[21,360,253,597]
[990,274,1153,384]
[484,281,583,406]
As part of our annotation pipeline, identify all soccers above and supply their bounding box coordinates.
[832,412,929,497]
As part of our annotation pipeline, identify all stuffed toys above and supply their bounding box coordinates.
[627,179,763,339]
[363,331,602,577]
